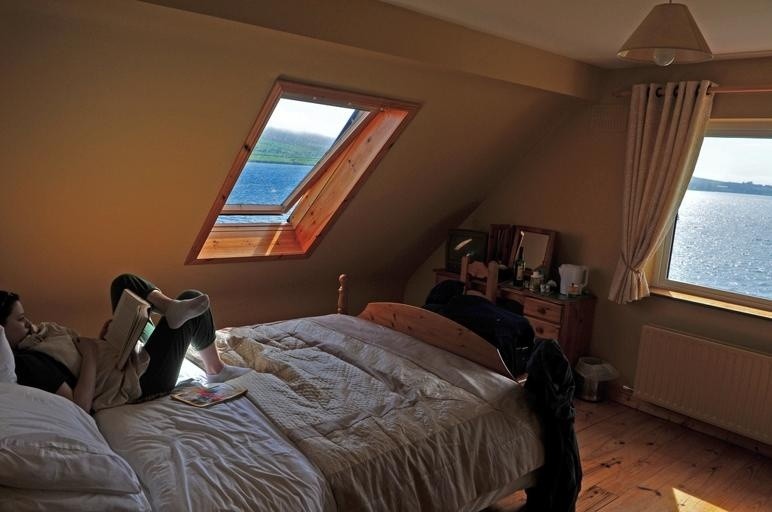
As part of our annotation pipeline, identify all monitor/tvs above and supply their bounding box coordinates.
[446,229,489,272]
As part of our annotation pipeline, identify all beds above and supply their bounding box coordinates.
[0,272,583,511]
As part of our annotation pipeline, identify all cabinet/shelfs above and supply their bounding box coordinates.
[430,266,598,369]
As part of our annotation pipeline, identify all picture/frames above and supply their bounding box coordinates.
[508,226,556,280]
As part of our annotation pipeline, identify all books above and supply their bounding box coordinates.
[170,382,248,408]
[102,288,151,371]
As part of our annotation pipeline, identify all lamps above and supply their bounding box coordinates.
[616,0,714,67]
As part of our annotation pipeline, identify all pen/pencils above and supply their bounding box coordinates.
[547,290,555,297]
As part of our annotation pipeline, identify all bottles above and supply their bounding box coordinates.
[529,271,544,291]
[513,247,525,287]
[566,279,582,297]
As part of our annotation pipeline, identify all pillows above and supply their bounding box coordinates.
[1,324,19,384]
[0,382,141,493]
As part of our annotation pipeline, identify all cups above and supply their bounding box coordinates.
[540,284,550,296]
[558,263,589,295]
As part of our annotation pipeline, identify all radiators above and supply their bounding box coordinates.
[622,321,772,456]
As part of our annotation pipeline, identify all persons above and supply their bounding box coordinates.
[0,273,253,417]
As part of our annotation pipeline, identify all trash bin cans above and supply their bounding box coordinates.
[575,356,610,403]
